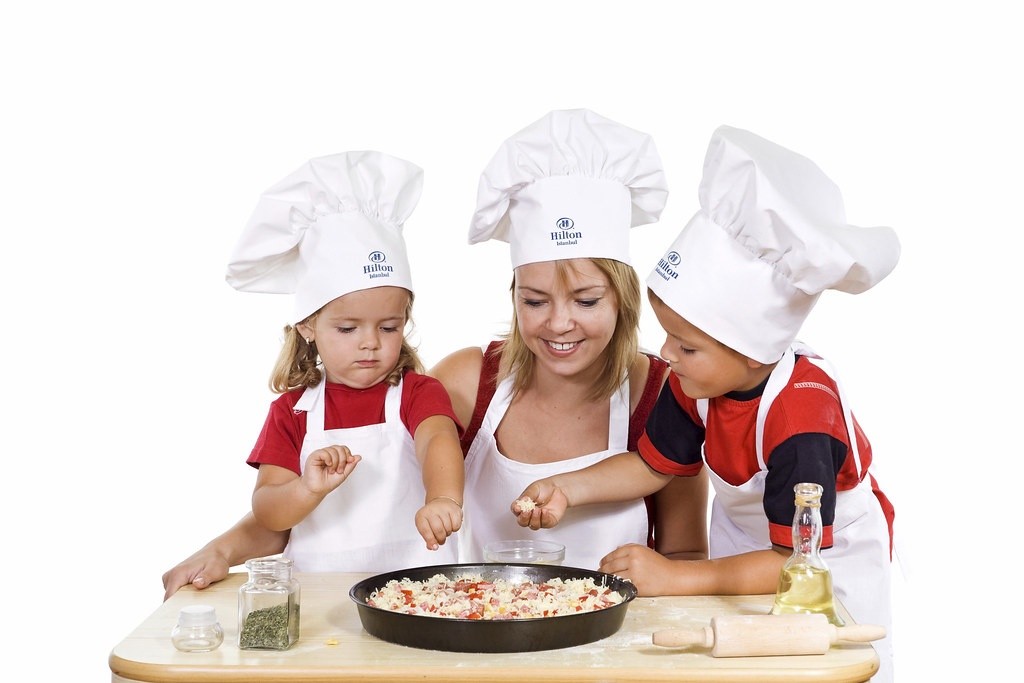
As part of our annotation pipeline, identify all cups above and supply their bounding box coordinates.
[482,539,566,567]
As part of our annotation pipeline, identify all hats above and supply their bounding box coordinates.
[226,151,423,328]
[469,109,668,272]
[649,125,900,364]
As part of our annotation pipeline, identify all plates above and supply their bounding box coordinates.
[350,563,638,653]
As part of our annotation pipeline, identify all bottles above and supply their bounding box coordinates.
[238,553,301,651]
[172,605,224,653]
[768,483,845,627]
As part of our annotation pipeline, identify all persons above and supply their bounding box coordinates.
[223,149,466,574]
[160,108,709,602]
[510,125,902,683]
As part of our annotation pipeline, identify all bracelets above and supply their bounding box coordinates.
[426,496,461,507]
